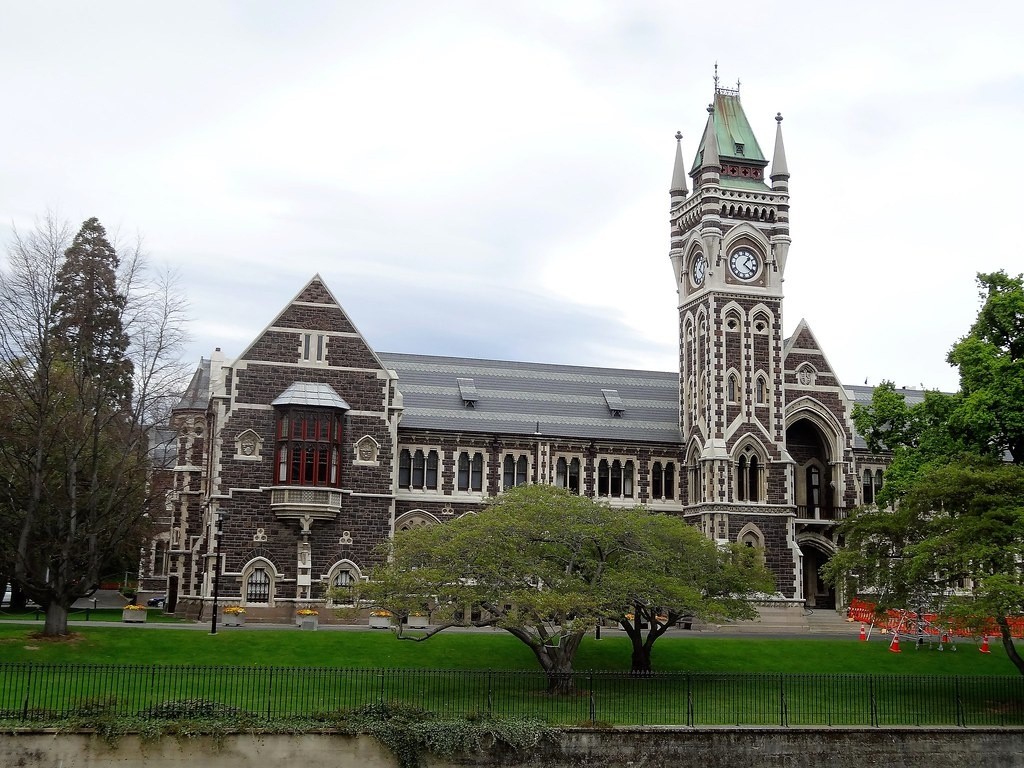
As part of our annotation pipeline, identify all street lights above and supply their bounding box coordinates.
[208,507,227,635]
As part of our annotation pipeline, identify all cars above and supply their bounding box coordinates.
[1,583,36,605]
[147,597,165,607]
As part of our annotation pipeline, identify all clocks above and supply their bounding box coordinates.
[689,250,705,287]
[728,244,763,282]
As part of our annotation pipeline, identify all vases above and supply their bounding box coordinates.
[369,616,391,629]
[296,614,318,627]
[618,619,635,630]
[408,616,429,629]
[648,620,667,629]
[122,609,147,622]
[222,613,245,625]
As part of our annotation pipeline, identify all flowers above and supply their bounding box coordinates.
[123,604,147,610]
[409,610,428,616]
[655,615,668,620]
[223,605,246,615]
[625,613,635,620]
[370,611,393,616]
[297,608,318,615]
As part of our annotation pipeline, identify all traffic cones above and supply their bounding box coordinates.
[860,623,867,641]
[942,635,949,643]
[890,631,902,653]
[979,632,991,654]
